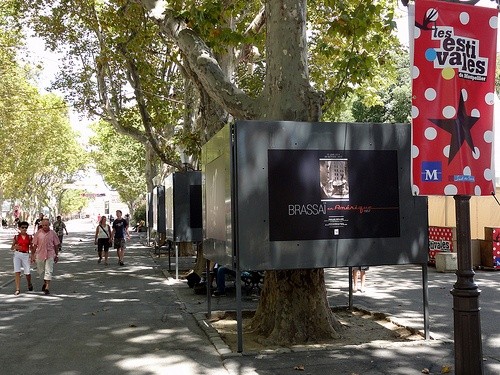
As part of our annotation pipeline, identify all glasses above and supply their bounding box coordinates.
[20,227,27,229]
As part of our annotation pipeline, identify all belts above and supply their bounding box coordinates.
[16,249,29,254]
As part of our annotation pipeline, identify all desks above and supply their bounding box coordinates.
[435,253,457,273]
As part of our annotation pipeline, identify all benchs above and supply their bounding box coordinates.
[155,240,174,257]
[240,270,265,295]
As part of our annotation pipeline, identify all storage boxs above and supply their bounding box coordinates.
[480,240,500,270]
[470,239,481,269]
[484,226,500,240]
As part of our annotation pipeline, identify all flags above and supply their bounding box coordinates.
[408,1,500,197]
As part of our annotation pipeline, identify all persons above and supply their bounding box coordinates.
[90,211,148,245]
[2,208,68,251]
[31,219,61,295]
[352,265,369,293]
[10,221,36,295]
[111,209,130,265]
[94,216,114,265]
[212,265,238,297]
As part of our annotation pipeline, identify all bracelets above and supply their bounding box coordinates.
[56,254,58,257]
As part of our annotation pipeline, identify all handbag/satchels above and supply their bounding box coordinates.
[109,239,112,247]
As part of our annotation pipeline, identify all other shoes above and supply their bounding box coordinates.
[212,292,226,298]
[42,285,46,291]
[14,290,20,295]
[27,284,33,291]
[45,288,50,294]
